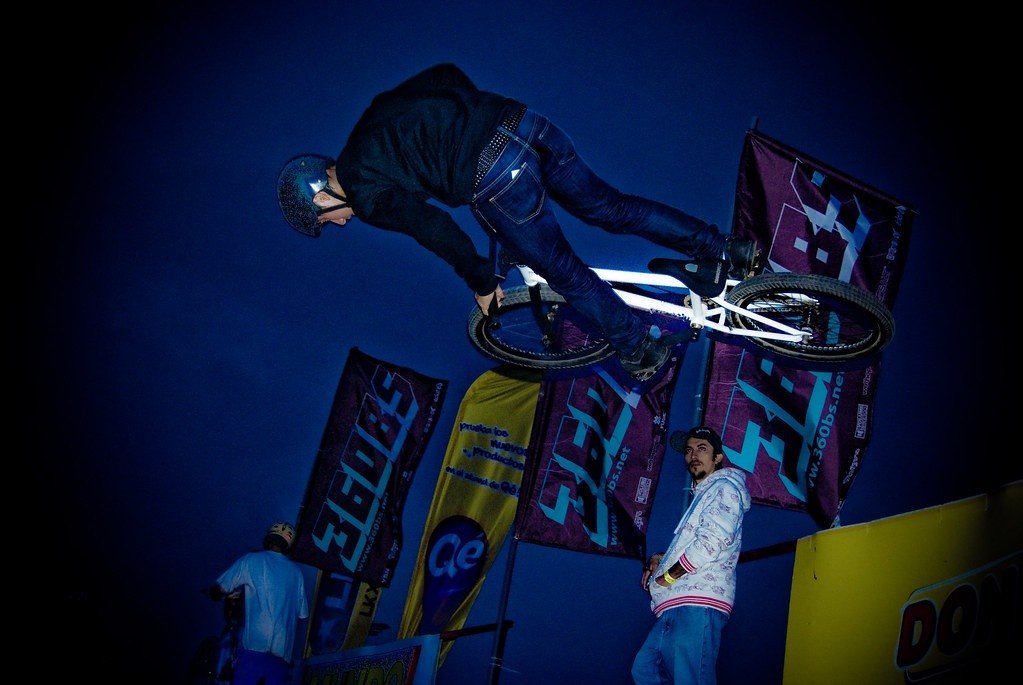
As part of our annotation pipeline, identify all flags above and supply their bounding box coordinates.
[512,303,689,565]
[691,131,920,530]
[285,346,449,592]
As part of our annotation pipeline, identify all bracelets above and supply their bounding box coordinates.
[664,570,677,584]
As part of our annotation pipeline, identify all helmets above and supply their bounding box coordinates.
[277,154,333,239]
[268,522,295,546]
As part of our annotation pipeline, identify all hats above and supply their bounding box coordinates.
[669,427,722,454]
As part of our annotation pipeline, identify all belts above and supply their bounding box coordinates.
[474,106,526,189]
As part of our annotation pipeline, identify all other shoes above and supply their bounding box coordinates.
[724,237,761,280]
[620,336,670,382]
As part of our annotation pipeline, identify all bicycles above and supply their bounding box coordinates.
[464,241,894,374]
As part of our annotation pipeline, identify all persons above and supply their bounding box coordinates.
[211,521,309,685]
[631,426,751,685]
[276,62,762,383]
